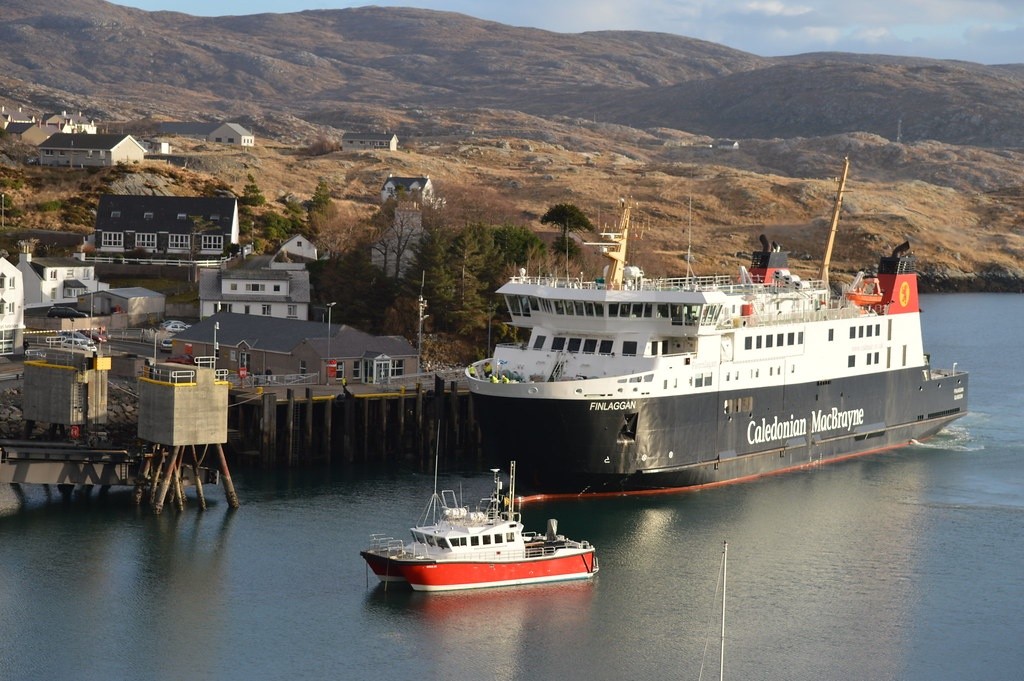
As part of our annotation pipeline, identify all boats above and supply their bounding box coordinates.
[359,454,601,594]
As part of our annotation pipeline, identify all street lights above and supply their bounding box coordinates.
[324,300,338,359]
[213,321,221,373]
[417,313,431,374]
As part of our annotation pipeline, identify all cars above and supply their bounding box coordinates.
[161,339,173,351]
[159,320,192,333]
[58,331,97,344]
[62,338,97,352]
[77,330,108,343]
[165,354,194,366]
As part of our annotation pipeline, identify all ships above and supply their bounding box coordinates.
[466,153,971,511]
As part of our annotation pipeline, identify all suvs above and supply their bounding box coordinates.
[47,307,90,318]
